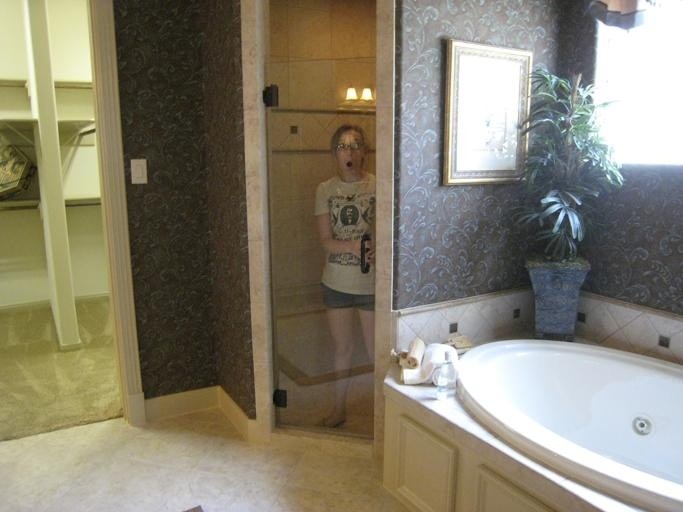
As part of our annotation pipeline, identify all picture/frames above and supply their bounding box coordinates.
[436,34,534,188]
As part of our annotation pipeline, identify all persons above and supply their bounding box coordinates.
[314,125,375,427]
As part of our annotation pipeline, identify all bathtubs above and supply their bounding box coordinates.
[458,339,683,512]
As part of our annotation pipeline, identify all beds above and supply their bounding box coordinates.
[503,65,627,340]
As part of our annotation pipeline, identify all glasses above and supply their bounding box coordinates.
[334,143,361,152]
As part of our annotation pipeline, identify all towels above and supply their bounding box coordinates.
[390,333,461,390]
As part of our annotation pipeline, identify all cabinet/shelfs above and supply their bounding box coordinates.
[0,78,107,210]
